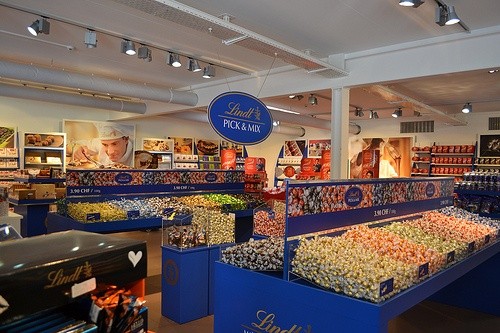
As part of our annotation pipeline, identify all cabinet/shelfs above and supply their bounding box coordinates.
[0,157,20,181]
[411,151,500,176]
[20,132,66,174]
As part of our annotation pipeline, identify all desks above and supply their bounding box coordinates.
[0,211,23,234]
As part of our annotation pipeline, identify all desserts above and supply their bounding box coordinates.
[27,134,55,146]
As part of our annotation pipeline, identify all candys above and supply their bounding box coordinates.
[66,171,245,186]
[292,206,500,302]
[55,193,286,271]
[288,179,455,217]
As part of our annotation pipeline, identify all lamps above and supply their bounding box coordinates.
[399,0,460,26]
[28,15,216,79]
[289,94,473,119]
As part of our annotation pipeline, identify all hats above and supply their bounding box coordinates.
[100,126,131,141]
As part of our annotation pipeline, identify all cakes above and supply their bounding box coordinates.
[198,140,218,154]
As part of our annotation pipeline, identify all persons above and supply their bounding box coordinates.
[366,170,373,178]
[313,158,321,172]
[256,158,264,171]
[75,124,134,167]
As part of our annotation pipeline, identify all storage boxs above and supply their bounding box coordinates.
[6,175,66,200]
